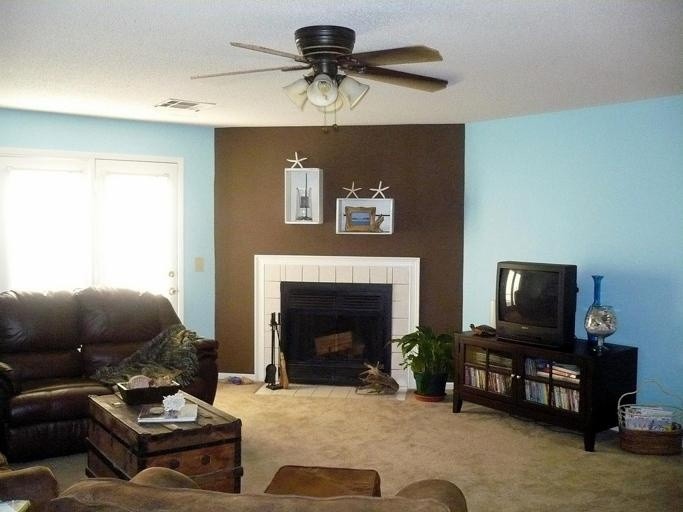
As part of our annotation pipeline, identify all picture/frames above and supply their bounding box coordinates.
[345,206,376,232]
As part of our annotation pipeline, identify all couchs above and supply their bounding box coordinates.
[0,288,219,463]
[47,467,467,512]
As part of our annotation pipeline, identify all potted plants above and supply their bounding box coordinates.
[385,325,454,402]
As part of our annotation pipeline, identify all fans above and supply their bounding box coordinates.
[190,25,448,93]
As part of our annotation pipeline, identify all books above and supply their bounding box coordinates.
[524,357,581,413]
[464,350,513,395]
[137,404,199,423]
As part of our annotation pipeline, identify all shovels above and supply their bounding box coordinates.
[264,313,279,385]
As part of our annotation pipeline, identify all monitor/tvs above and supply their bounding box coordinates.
[495,261,579,351]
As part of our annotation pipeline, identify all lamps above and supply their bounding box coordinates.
[281,72,370,113]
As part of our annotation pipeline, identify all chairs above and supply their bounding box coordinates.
[0,454,58,511]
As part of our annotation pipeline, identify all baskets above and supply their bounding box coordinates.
[618,421,683,455]
[617,379,683,432]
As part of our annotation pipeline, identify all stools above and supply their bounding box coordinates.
[263,465,381,498]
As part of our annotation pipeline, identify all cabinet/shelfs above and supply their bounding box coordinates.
[283,164,324,225]
[335,197,395,235]
[455,331,637,434]
